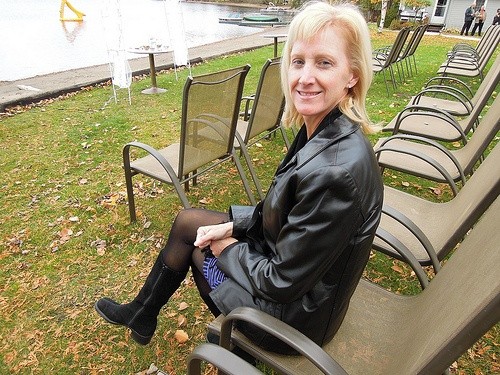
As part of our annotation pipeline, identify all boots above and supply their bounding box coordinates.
[202,295,258,367]
[95,250,189,346]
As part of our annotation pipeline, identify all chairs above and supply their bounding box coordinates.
[372,23,427,97]
[431,22,500,99]
[186,54,500,375]
[122,57,299,222]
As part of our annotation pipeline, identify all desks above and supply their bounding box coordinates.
[128,47,175,94]
[264,34,288,58]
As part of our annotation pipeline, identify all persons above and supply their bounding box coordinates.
[96,3,383,356]
[493,9,500,25]
[460,3,476,36]
[471,5,487,36]
